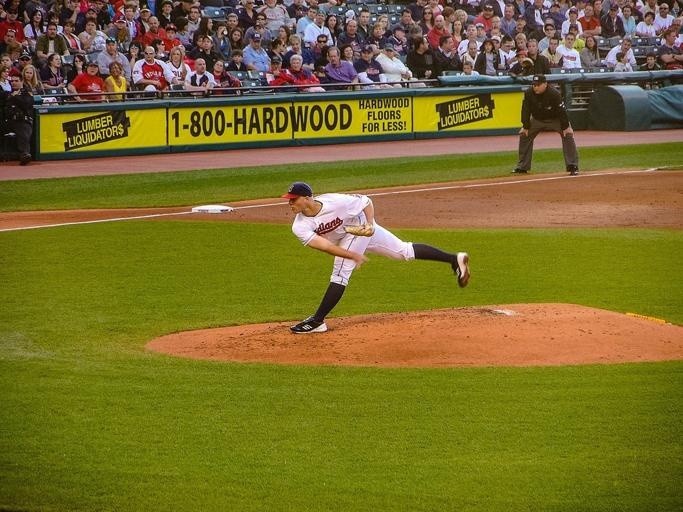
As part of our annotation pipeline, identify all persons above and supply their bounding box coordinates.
[1,0,683,109]
[510,74,579,176]
[0,71,34,165]
[277,181,470,334]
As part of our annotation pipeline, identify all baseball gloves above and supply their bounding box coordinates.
[343,222,376,239]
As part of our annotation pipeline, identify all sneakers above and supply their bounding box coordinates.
[289,315,328,334]
[451,252,470,288]
[19,154,32,165]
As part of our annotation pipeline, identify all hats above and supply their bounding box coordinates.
[531,75,546,85]
[252,32,261,42]
[361,24,409,70]
[476,3,618,56]
[345,10,355,24]
[20,51,32,60]
[166,24,177,32]
[106,37,116,45]
[271,56,282,65]
[281,182,312,199]
[140,4,151,13]
[7,5,19,13]
[88,59,99,67]
[116,16,127,23]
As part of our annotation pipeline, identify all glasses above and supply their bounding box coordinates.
[144,51,153,56]
[6,28,16,34]
[424,11,431,15]
[660,7,668,10]
[317,40,326,44]
[159,43,165,47]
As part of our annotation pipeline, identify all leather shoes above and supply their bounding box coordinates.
[569,166,578,175]
[511,167,527,174]
